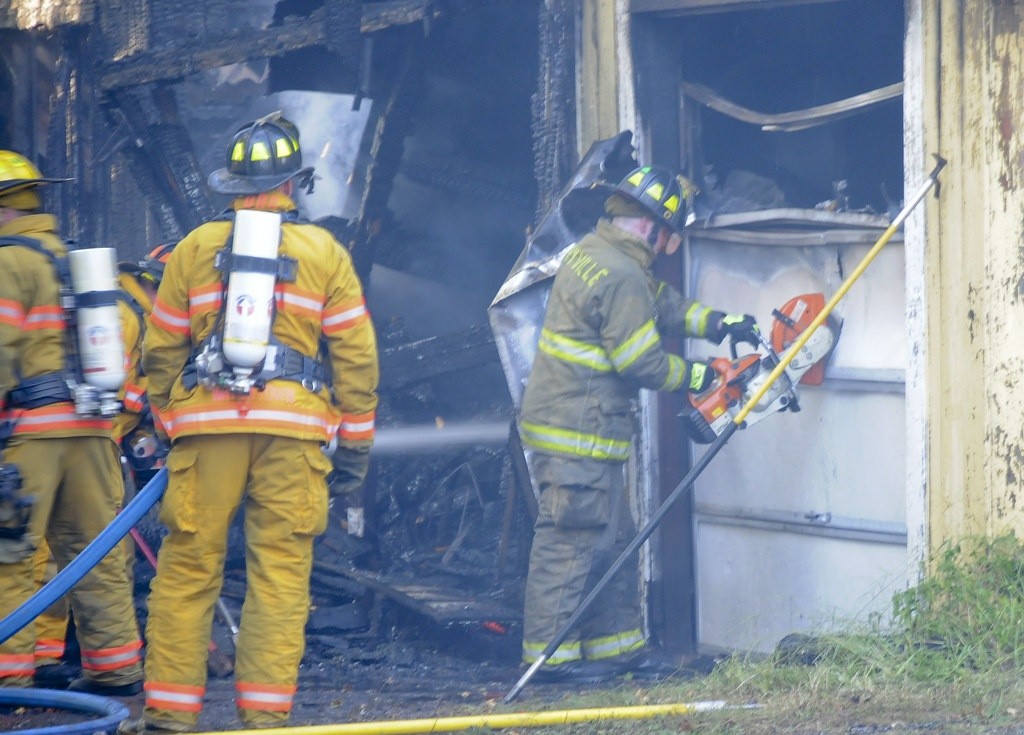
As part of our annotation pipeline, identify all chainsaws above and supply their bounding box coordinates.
[678,293,844,445]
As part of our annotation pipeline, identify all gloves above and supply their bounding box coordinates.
[688,360,715,394]
[325,445,372,497]
[152,439,171,458]
[705,311,761,351]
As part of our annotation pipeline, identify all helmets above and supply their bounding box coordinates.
[0,149,75,192]
[206,119,316,195]
[614,164,701,237]
[116,242,179,272]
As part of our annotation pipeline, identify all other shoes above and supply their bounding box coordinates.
[33,663,83,688]
[607,637,696,683]
[516,655,619,685]
[66,676,144,696]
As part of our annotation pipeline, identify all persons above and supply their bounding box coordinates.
[0,151,143,693]
[118,121,378,735]
[33,242,183,686]
[518,165,760,682]
